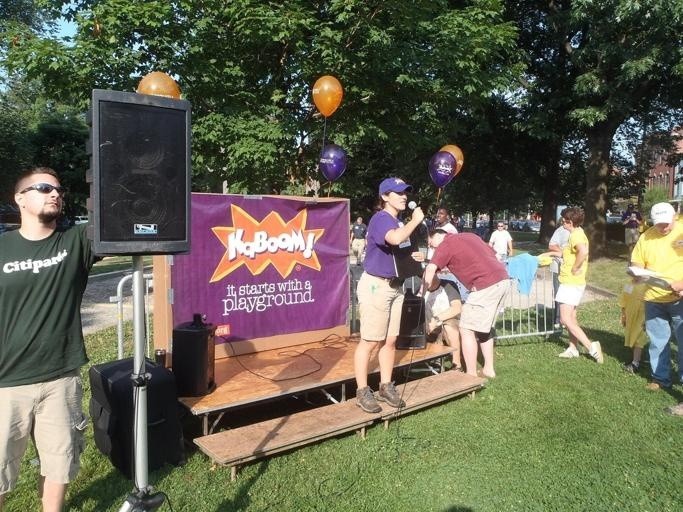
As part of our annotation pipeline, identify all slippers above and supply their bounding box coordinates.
[477,367,494,382]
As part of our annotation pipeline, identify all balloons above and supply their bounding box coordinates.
[310,75,343,117]
[427,150,456,189]
[437,144,464,178]
[135,70,179,99]
[323,145,346,180]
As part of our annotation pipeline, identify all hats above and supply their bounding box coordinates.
[378,177,413,194]
[628,204,634,208]
[651,202,675,225]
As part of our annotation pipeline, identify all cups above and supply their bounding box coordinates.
[155,348,165,366]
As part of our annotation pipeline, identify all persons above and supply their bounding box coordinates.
[0,165,103,512]
[353,176,427,413]
[549,206,604,364]
[349,217,368,265]
[399,207,513,378]
[617,203,683,390]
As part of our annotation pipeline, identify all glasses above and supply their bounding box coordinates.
[20,183,65,197]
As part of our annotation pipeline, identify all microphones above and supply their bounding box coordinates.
[408,200,427,227]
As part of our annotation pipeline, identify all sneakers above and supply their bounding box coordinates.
[559,348,578,357]
[589,341,603,364]
[357,387,382,412]
[645,382,672,393]
[664,402,683,416]
[622,363,640,373]
[377,383,406,407]
[431,356,465,373]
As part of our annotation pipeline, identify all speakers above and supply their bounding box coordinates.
[396,298,425,350]
[173,322,217,397]
[92,89,190,254]
[88,356,178,480]
